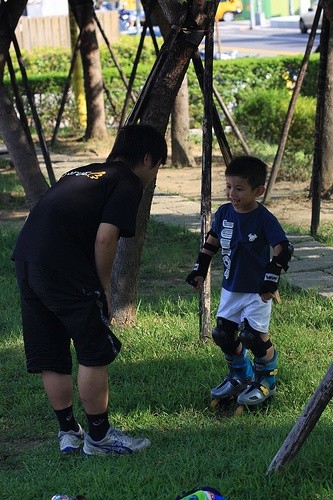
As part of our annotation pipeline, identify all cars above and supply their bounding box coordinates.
[298,0,325,34]
[215,0,245,21]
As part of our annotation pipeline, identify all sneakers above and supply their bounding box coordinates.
[83,425,152,456]
[58,422,86,453]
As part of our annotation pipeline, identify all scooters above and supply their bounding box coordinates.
[120,13,131,28]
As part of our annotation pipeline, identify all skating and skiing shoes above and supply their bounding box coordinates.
[235,350,278,415]
[210,345,255,409]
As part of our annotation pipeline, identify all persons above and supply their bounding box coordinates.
[10,123,166,456]
[186,156,294,406]
